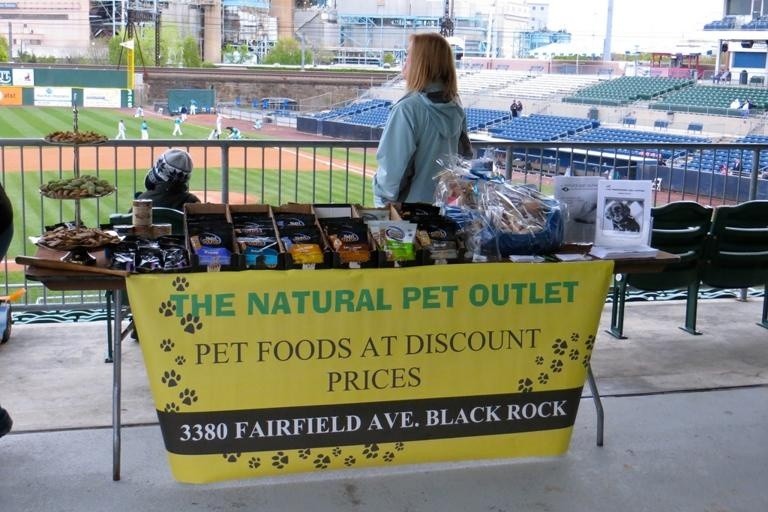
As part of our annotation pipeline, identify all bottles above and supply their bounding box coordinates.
[111,198,172,237]
[0,296,12,346]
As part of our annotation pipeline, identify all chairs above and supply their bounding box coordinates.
[8,303,133,325]
[698,197,768,332]
[705,11,768,30]
[602,199,714,342]
[313,98,537,145]
[537,70,768,178]
[388,71,537,98]
[103,205,188,367]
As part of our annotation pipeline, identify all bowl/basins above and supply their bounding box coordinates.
[561,241,595,254]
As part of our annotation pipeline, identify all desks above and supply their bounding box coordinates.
[14,253,608,484]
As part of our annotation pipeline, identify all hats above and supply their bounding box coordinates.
[144,149,194,192]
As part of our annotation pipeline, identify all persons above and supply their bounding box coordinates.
[0,183,14,440]
[719,157,768,179]
[115,103,262,140]
[564,164,575,176]
[730,98,750,109]
[523,159,532,171]
[713,68,729,84]
[510,100,523,117]
[657,153,665,165]
[373,32,473,209]
[112,148,201,340]
